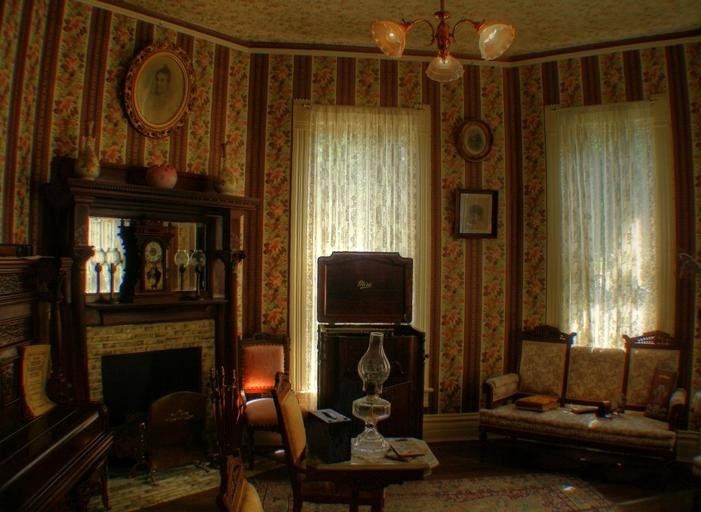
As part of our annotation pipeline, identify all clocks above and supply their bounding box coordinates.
[118,212,177,302]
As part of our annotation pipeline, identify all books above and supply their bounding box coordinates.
[386,437,426,463]
[514,393,560,413]
[20,348,57,419]
[644,367,678,422]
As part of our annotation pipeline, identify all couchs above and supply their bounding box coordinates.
[478,325,687,490]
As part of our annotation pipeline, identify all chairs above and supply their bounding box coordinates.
[271,371,386,512]
[237,330,291,468]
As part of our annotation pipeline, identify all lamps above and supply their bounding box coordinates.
[371,5,516,81]
[352,332,390,459]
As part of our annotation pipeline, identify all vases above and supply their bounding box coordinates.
[216,142,237,194]
[78,135,101,180]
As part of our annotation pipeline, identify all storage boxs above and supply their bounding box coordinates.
[316,251,425,441]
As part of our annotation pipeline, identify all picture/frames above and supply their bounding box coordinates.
[124,39,199,139]
[455,121,494,162]
[455,189,498,240]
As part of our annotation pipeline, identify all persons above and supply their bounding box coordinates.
[465,204,488,231]
